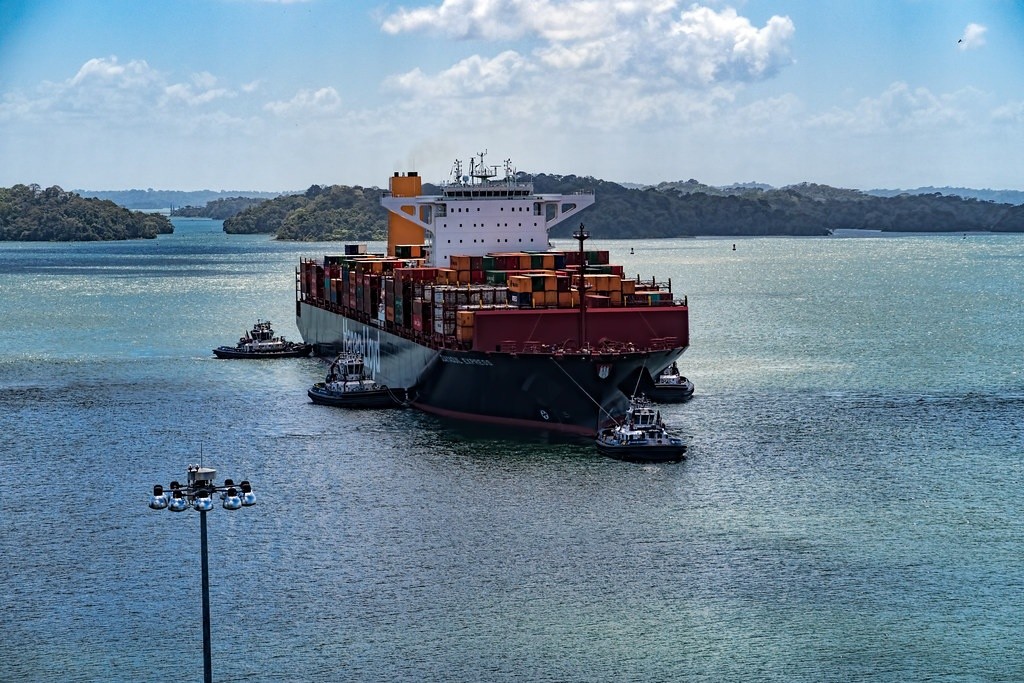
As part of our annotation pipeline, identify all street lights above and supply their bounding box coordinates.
[145,448,261,683]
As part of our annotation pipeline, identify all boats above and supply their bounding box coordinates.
[592,391,690,463]
[646,362,695,404]
[306,349,408,410]
[212,318,307,362]
[293,150,692,446]
